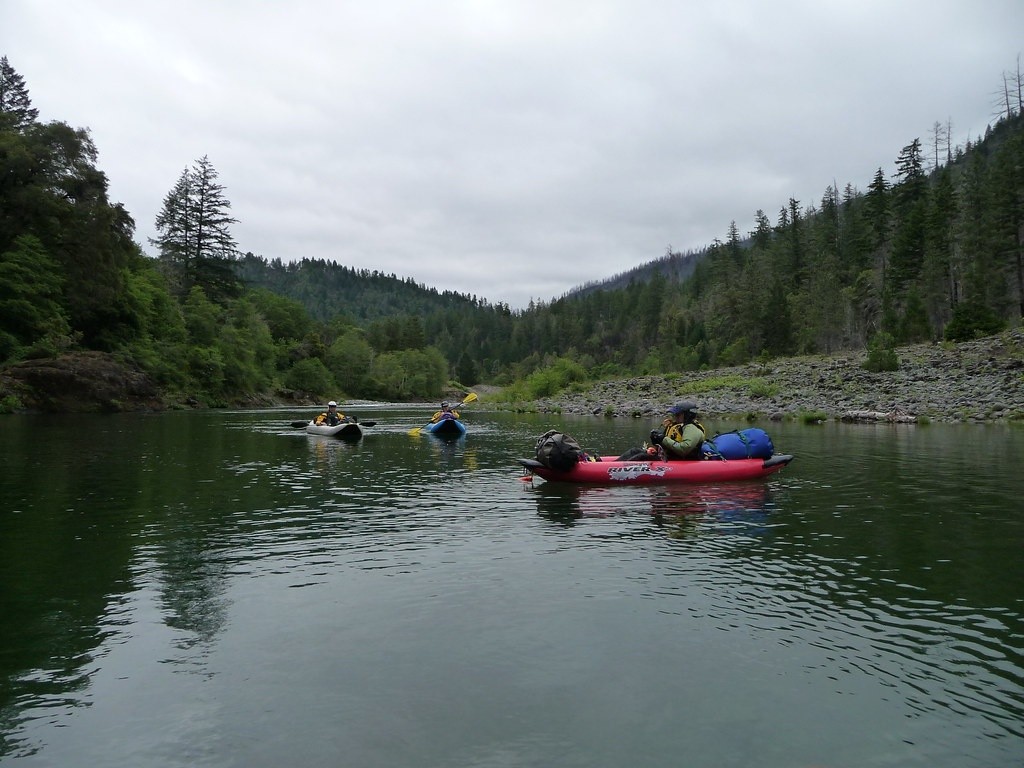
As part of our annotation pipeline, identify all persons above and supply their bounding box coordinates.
[429,401,459,423]
[594,402,706,461]
[316,400,347,426]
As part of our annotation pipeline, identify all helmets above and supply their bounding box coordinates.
[665,401,696,424]
[328,401,336,410]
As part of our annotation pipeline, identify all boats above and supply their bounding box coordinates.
[425,418,466,435]
[518,453,795,484]
[306,415,363,436]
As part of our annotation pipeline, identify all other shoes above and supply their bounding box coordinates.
[594,453,602,462]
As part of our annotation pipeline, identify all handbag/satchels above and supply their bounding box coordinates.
[536,430,581,472]
[699,428,774,460]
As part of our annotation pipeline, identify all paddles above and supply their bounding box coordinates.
[409,392,478,436]
[290,421,377,428]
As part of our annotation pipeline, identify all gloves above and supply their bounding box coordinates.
[650,429,665,446]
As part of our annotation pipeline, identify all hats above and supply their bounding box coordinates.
[441,401,449,407]
